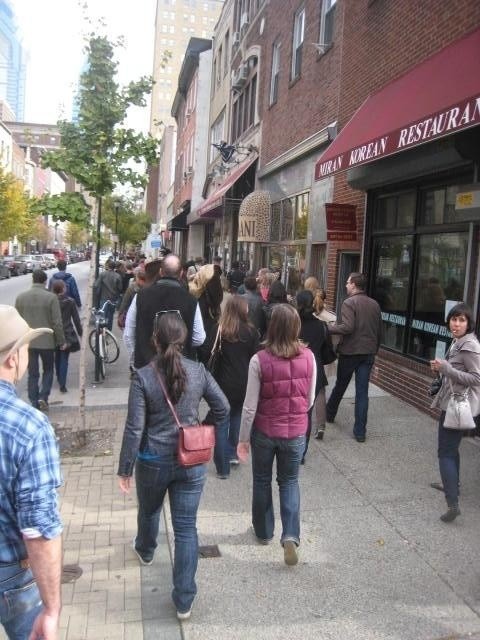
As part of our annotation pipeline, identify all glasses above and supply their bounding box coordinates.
[156,310,182,331]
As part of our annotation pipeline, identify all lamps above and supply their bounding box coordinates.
[208,140,256,177]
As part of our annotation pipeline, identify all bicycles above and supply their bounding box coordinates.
[88,300,120,381]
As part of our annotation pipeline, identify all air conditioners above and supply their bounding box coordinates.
[233,32,240,46]
[232,63,248,90]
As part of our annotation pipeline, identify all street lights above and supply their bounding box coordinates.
[114,199,121,263]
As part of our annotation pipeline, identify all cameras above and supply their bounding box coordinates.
[426,377,442,395]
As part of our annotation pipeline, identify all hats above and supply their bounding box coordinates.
[194,256,204,263]
[0,305,54,366]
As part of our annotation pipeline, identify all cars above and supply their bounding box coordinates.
[0,246,111,279]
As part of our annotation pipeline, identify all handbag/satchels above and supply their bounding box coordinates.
[59,328,80,352]
[118,312,126,327]
[443,396,476,429]
[205,349,226,384]
[322,341,337,364]
[179,425,215,466]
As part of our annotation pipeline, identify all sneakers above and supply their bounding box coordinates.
[284,540,298,564]
[354,430,365,442]
[177,600,193,619]
[431,482,445,491]
[60,386,67,392]
[215,472,230,479]
[133,537,154,565]
[326,412,335,423]
[252,524,273,544]
[440,506,460,522]
[37,393,48,411]
[314,424,325,438]
[230,456,239,464]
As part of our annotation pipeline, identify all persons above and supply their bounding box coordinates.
[16,252,382,479]
[117,308,232,620]
[429,303,479,522]
[372,272,462,360]
[235,304,317,566]
[0,304,64,639]
